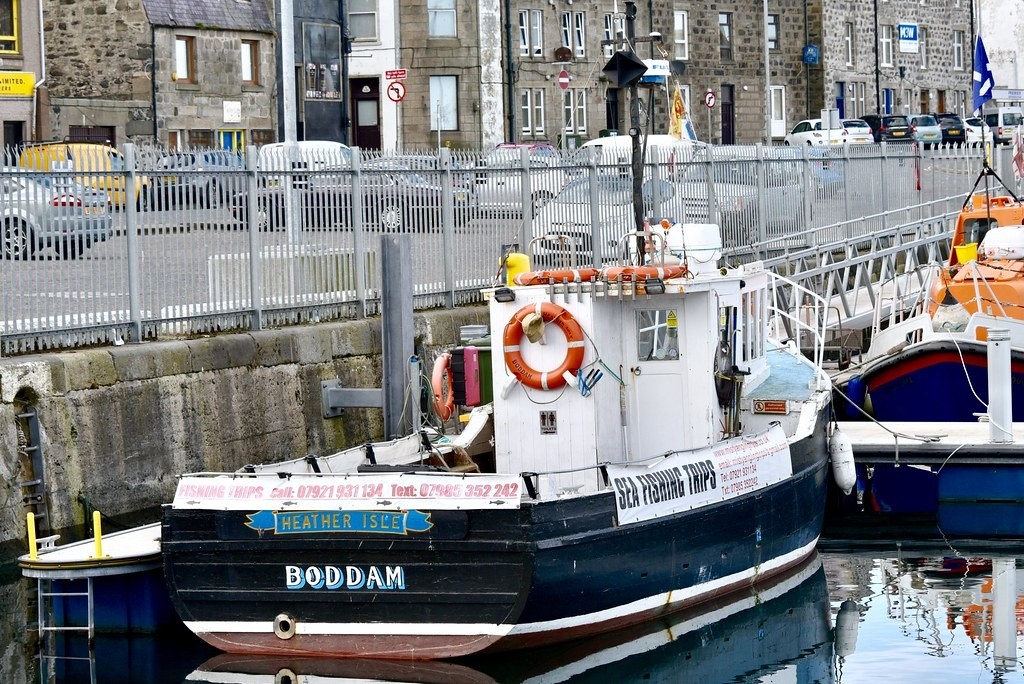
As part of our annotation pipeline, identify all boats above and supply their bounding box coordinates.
[925,159,1023,332]
[155,219,837,659]
[172,545,838,684]
[792,257,1024,542]
[9,501,176,642]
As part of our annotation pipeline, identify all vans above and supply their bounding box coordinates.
[982,106,1024,148]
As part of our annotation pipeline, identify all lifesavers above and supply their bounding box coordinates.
[430,354,457,422]
[502,300,585,393]
[514,263,686,287]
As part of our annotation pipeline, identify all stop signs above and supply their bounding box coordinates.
[557,70,570,89]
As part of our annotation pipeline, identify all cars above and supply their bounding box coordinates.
[531,174,687,265]
[1,165,113,261]
[228,134,846,248]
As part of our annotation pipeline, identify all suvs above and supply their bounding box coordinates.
[17,134,153,212]
[962,117,993,144]
[150,151,266,210]
[858,113,917,150]
[921,111,968,148]
[783,117,875,158]
[906,114,943,144]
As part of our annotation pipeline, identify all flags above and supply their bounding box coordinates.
[973,30,995,118]
[668,87,689,139]
